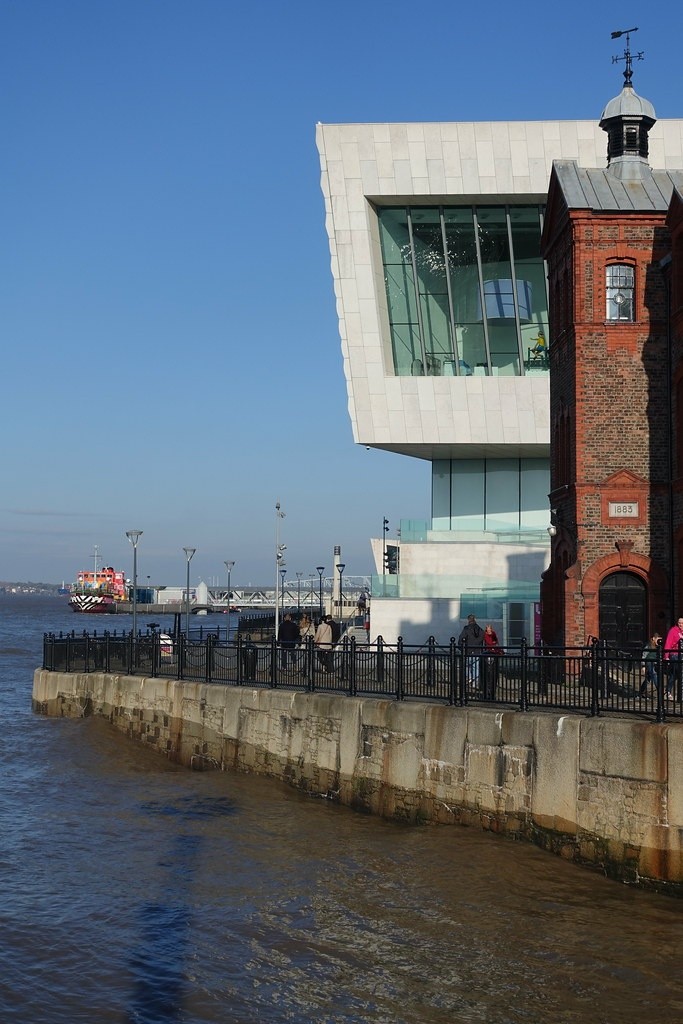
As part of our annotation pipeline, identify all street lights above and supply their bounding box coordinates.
[336,564,346,636]
[183,547,196,654]
[125,529,144,668]
[316,566,325,621]
[272,495,287,642]
[381,516,389,595]
[224,560,235,645]
[278,569,286,622]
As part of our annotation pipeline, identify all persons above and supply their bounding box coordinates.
[636,631,663,699]
[325,615,341,673]
[278,613,302,673]
[298,612,315,671]
[316,615,331,673]
[457,613,485,687]
[663,617,682,702]
[357,592,366,612]
[485,625,505,655]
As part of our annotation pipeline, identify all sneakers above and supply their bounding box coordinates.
[664,693,675,701]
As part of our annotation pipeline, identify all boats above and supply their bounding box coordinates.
[67,544,240,616]
